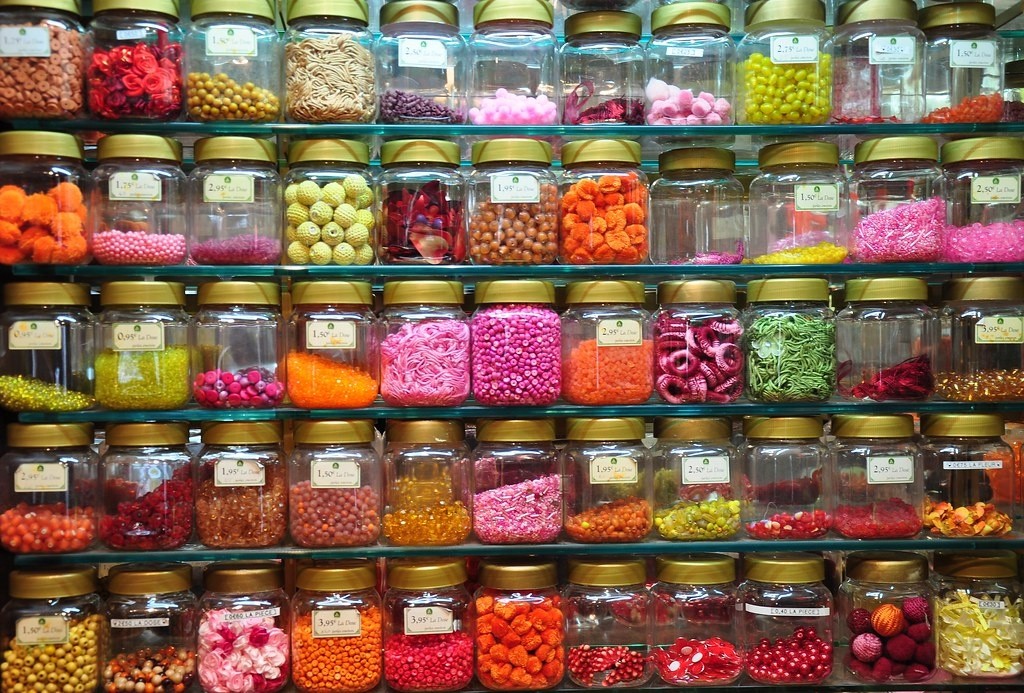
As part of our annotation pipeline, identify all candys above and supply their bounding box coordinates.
[1,19,1024,692]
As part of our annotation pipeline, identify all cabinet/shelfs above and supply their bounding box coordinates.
[0,118,1024,693]
